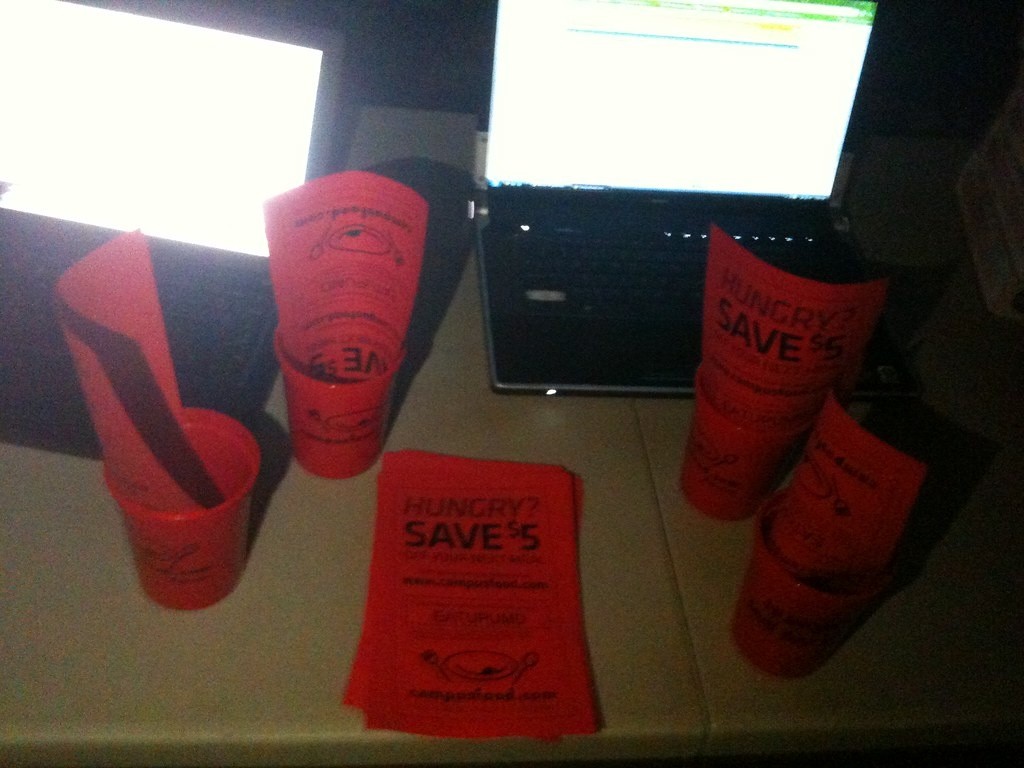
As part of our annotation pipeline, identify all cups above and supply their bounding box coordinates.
[103,407,260,609]
[680,362,814,522]
[273,323,404,480]
[730,502,883,680]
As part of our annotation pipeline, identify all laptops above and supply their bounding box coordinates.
[470,0,880,398]
[0,0,346,463]
